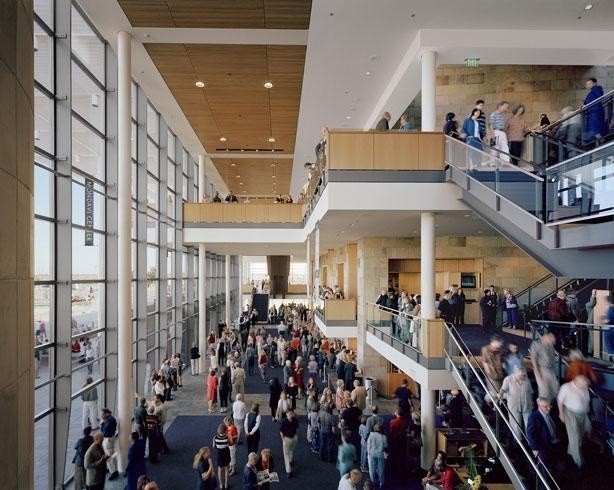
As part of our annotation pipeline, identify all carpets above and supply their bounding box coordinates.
[240,325,335,395]
[126,412,419,490]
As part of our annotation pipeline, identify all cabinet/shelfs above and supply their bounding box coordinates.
[385,361,415,398]
[437,429,488,466]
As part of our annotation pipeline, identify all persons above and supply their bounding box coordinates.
[211,190,221,203]
[225,191,238,204]
[443,78,606,166]
[199,193,209,203]
[399,114,413,131]
[274,127,328,204]
[35,279,613,490]
[375,112,391,131]
